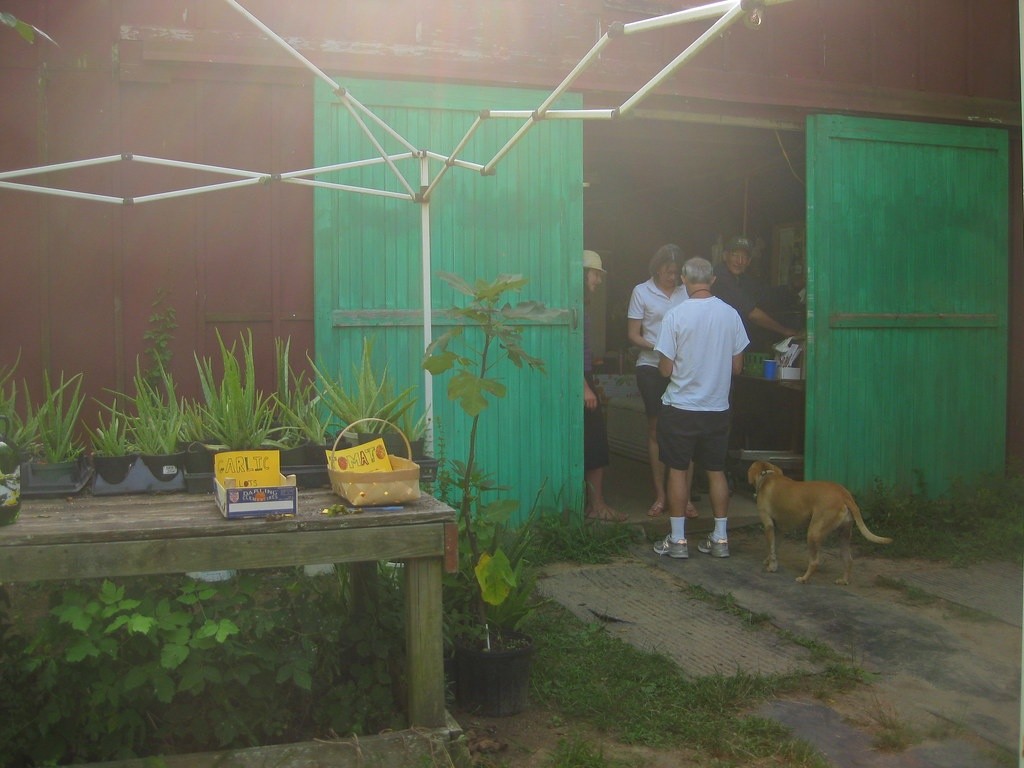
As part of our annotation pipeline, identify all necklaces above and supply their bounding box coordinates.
[689,289,713,298]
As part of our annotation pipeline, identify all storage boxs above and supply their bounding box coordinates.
[212,473,298,520]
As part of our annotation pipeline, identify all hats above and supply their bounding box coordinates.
[584,250,606,273]
[724,235,751,254]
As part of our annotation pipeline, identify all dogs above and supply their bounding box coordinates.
[747,460,895,586]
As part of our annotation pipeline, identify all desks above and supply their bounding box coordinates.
[734,373,804,467]
[1,482,459,731]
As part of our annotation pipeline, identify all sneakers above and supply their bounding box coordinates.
[697,533,729,557]
[654,534,690,558]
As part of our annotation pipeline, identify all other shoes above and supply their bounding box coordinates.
[586,500,627,521]
[685,502,698,518]
[647,501,664,515]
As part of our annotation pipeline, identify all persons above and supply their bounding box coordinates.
[653,257,751,558]
[628,244,699,517]
[712,236,806,496]
[583,250,629,521]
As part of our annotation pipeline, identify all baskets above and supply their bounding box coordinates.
[742,351,773,376]
[327,417,421,508]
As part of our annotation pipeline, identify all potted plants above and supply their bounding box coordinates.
[0,324,438,492]
[419,254,572,718]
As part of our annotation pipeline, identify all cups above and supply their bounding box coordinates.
[763,360,777,378]
